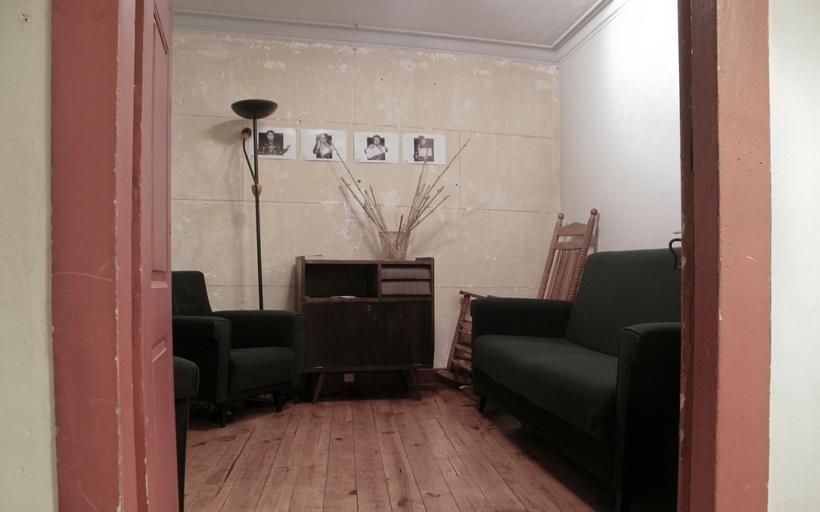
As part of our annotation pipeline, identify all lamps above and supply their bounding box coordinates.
[230,99,279,310]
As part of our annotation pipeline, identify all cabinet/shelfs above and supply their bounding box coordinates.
[294,254,435,404]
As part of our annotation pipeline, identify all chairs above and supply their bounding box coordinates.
[435,208,598,392]
[171,270,300,428]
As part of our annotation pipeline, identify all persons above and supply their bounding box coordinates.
[257,130,291,156]
[413,136,434,162]
[312,133,337,159]
[363,135,387,160]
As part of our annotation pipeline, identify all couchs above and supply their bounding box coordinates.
[468,246,681,511]
